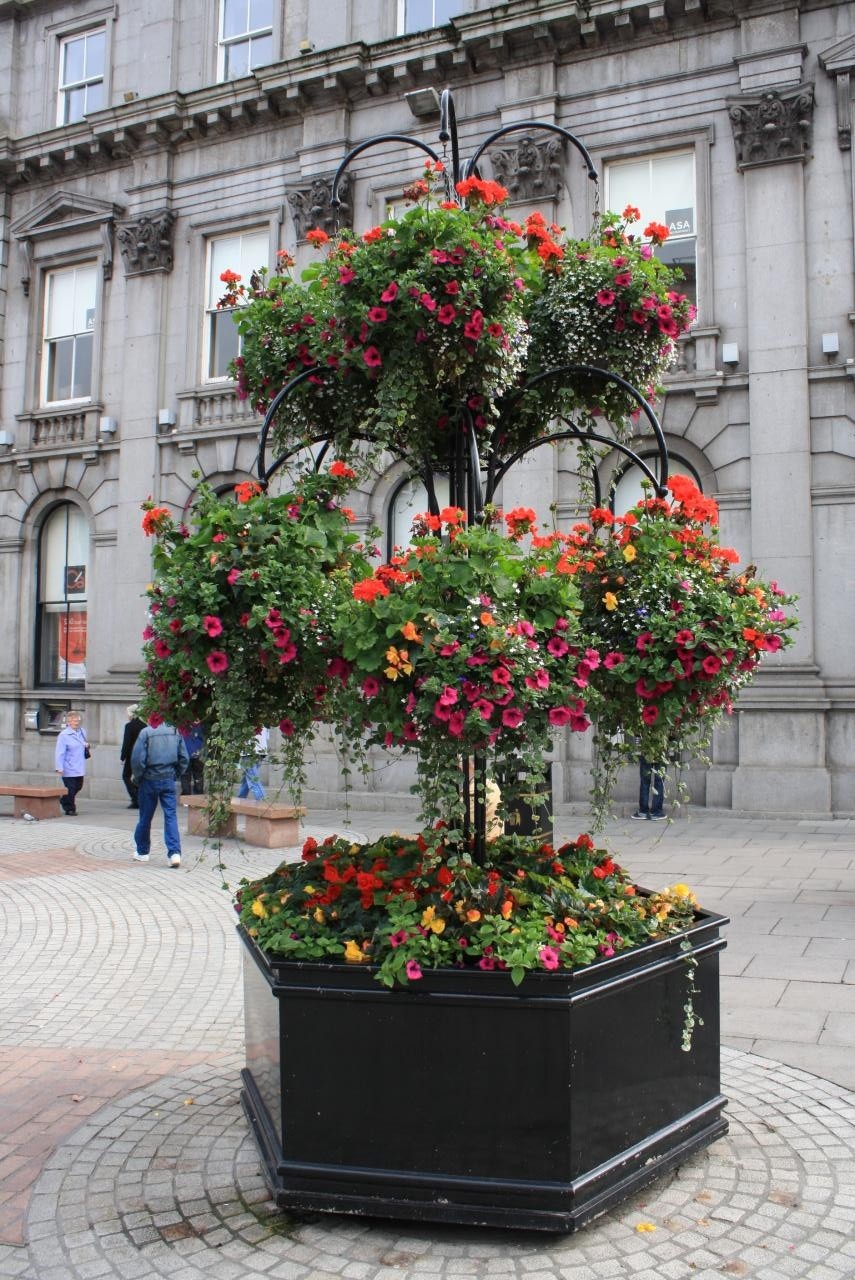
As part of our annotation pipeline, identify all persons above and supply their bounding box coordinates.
[237,727,269,799]
[55,711,90,816]
[631,734,674,819]
[131,722,189,867]
[181,717,203,795]
[120,704,148,810]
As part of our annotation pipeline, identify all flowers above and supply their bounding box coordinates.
[134,458,804,900]
[231,819,706,1051]
[217,159,699,515]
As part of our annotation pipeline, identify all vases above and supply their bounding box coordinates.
[240,885,730,1232]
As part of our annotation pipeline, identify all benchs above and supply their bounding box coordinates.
[178,795,307,849]
[0,784,69,820]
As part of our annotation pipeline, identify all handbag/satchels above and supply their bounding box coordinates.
[84,745,91,759]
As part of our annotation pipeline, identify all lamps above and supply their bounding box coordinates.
[404,86,442,116]
[124,92,139,103]
[298,40,315,54]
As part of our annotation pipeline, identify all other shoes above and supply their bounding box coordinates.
[134,851,149,862]
[167,854,181,868]
[651,814,667,821]
[65,810,77,816]
[631,810,648,820]
[60,796,67,810]
